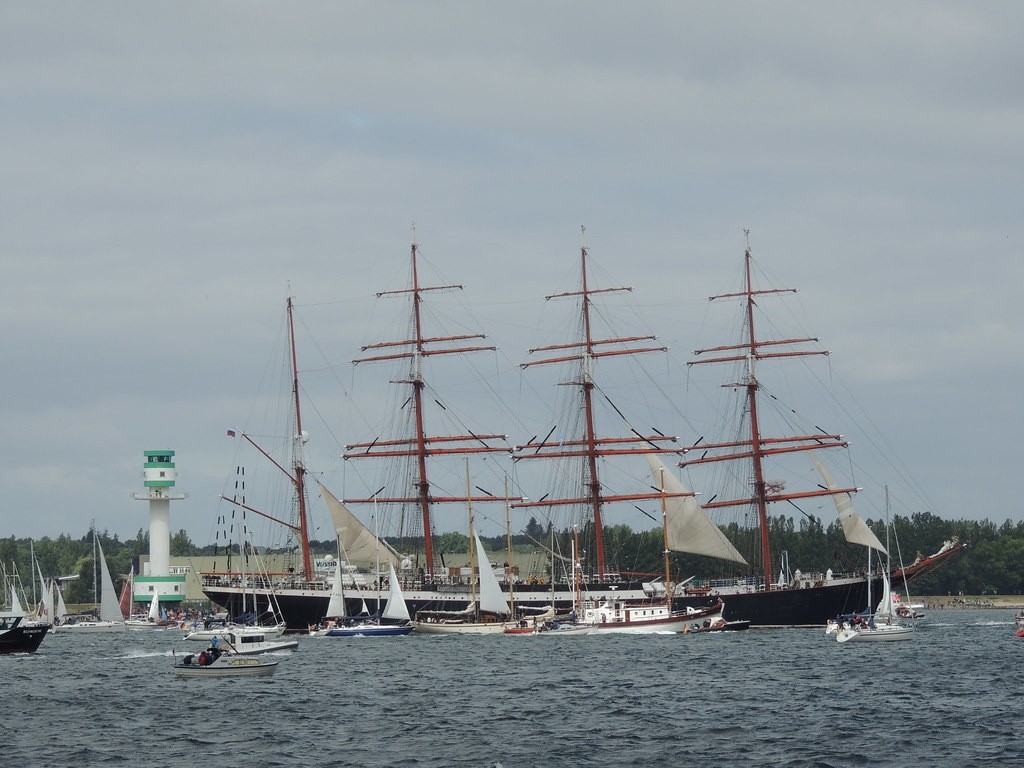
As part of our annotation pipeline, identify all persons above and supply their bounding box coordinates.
[831,588,995,630]
[795,568,834,580]
[145,560,765,668]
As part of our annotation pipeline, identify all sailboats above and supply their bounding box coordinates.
[201,213,964,623]
[824,478,926,642]
[0,422,728,654]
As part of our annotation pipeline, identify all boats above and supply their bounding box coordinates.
[206,640,302,656]
[169,642,282,679]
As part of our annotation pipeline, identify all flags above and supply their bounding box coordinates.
[227,430,235,436]
